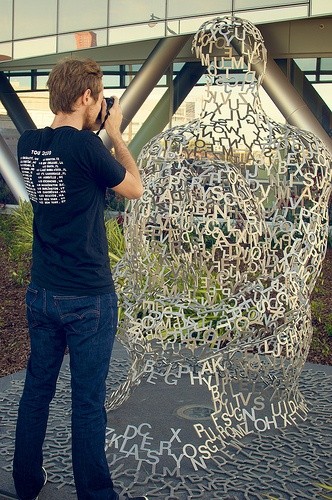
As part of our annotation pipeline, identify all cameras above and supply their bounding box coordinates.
[97,98,114,119]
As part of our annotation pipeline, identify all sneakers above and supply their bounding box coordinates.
[35,467,47,500]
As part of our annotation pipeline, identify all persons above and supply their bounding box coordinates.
[11,55,145,500]
